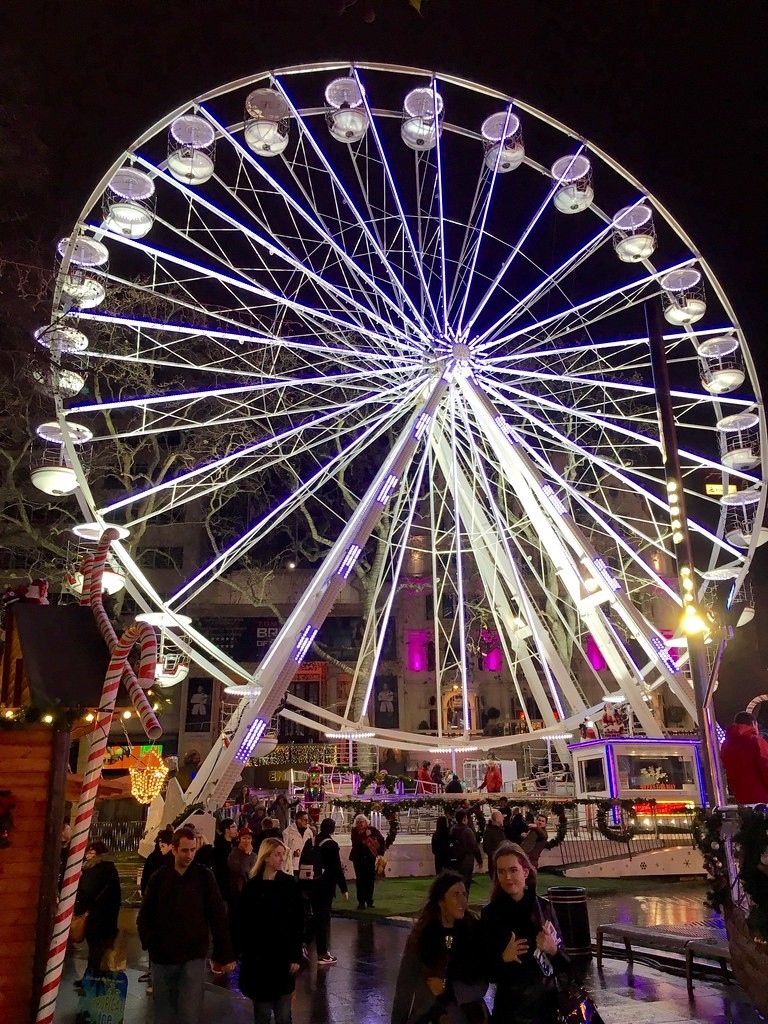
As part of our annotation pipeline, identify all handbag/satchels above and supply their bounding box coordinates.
[554,988,605,1024]
[375,854,386,880]
[69,910,90,943]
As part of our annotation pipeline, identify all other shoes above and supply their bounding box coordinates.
[357,906,365,910]
[367,904,374,908]
[316,951,337,964]
[74,980,82,987]
[209,958,222,973]
[139,973,152,982]
[302,946,312,960]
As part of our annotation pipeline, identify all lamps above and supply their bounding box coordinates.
[129,739,169,805]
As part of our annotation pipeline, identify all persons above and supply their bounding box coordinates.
[378,682,393,728]
[718,710,768,806]
[189,684,208,722]
[56,759,567,1024]
[602,702,624,738]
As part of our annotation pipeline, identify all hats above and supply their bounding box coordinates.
[239,828,253,838]
[92,840,108,855]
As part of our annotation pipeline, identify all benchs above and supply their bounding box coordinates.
[596,922,732,991]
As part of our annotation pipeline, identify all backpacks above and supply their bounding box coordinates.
[446,825,465,857]
[299,837,332,880]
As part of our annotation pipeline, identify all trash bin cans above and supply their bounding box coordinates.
[547,886,593,962]
